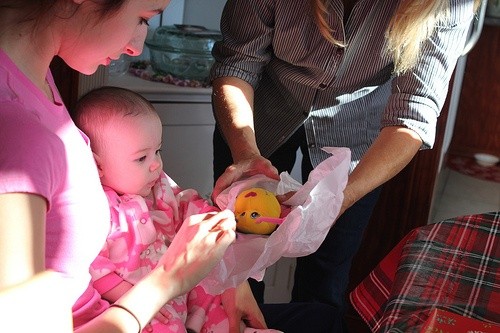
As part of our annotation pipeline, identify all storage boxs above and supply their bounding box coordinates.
[144,25,223,81]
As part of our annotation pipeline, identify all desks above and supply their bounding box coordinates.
[350,211,500,333]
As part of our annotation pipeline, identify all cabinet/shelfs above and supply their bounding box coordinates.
[105,74,303,304]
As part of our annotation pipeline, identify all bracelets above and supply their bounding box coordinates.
[105,302,141,333]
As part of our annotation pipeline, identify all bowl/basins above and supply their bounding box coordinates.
[473,154,500,167]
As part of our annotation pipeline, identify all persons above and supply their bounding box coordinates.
[70,86,284,333]
[0,0,268,333]
[211,0,482,333]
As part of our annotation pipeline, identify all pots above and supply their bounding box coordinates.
[147,24,223,79]
